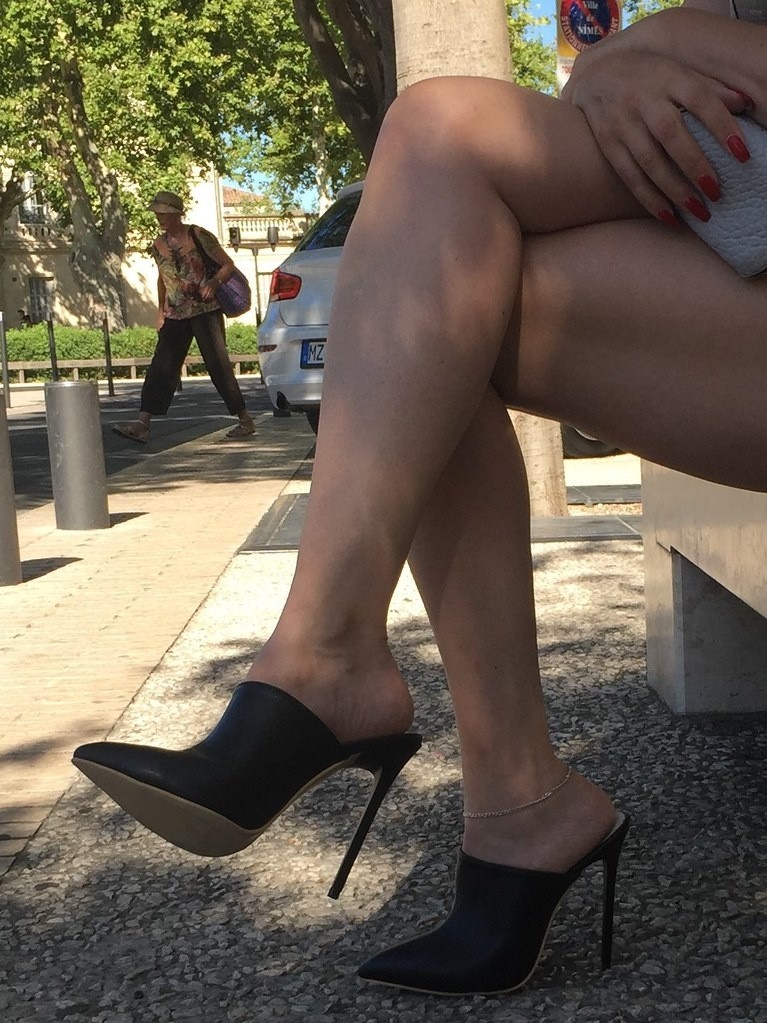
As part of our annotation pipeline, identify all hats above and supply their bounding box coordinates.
[145,191,186,216]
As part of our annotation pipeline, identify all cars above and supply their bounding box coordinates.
[259,175,371,438]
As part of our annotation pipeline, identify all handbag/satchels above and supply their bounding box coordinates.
[218,266,252,318]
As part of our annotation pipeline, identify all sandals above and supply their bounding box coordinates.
[113,424,151,444]
[225,416,257,437]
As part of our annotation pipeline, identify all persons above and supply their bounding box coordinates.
[112,190,257,443]
[65,0,767,997]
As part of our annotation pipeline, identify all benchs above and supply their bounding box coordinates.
[639,457,767,719]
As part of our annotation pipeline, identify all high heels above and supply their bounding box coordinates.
[75,683,627,998]
